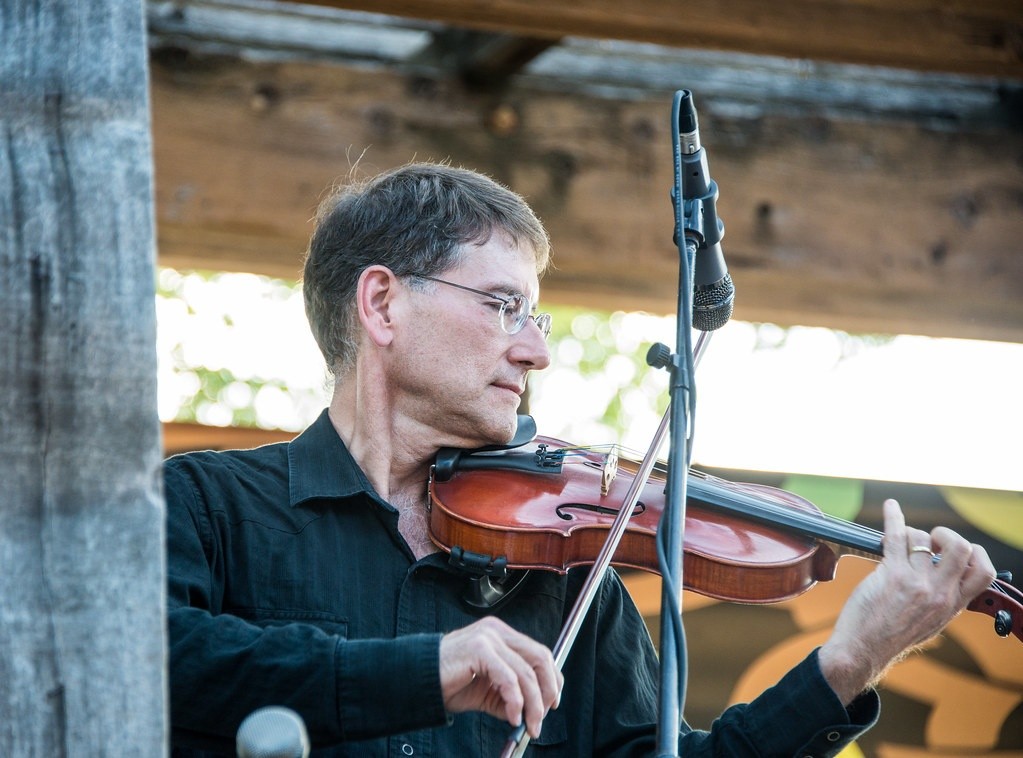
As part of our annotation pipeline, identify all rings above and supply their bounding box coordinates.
[909,547,942,564]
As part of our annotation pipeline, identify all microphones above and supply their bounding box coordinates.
[677,88,735,332]
[237,704,310,758]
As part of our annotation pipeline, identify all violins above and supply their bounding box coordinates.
[424,413,1023,644]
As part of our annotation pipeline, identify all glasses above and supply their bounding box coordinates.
[406,271,551,341]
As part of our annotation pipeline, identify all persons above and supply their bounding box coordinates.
[162,163,996,758]
